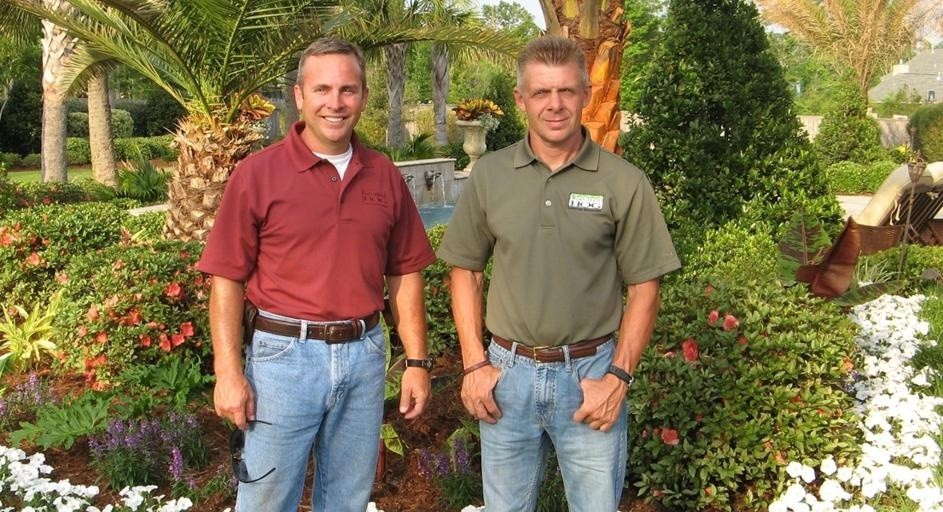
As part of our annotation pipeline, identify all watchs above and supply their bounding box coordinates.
[403,355,434,376]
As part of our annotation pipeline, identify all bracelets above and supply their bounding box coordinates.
[462,358,490,373]
[606,362,636,391]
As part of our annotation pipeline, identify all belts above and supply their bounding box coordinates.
[491,334,614,362]
[254,309,381,344]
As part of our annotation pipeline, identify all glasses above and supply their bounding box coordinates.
[228,419,276,483]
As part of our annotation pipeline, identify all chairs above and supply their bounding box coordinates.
[855,159,943,235]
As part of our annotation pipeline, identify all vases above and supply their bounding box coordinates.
[455,118,487,173]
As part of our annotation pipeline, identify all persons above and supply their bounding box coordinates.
[434,29,683,511]
[195,34,436,511]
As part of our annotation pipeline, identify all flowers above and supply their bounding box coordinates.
[450,97,503,131]
